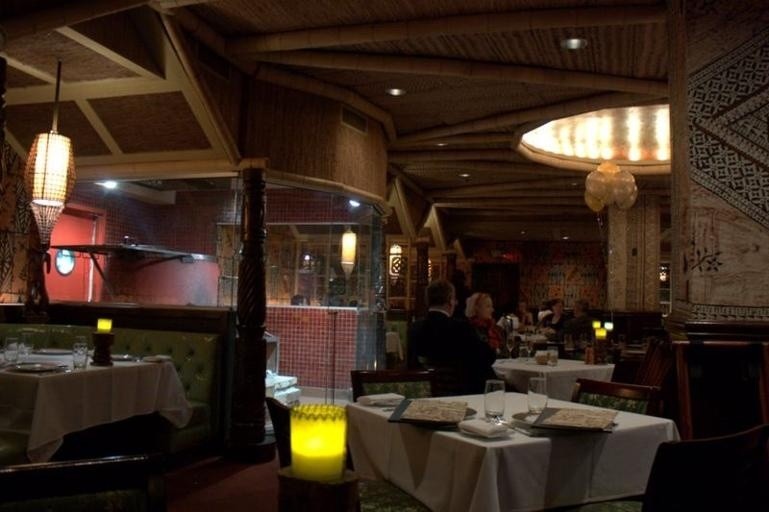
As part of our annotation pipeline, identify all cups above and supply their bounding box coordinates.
[526,377,548,415]
[535,350,559,366]
[5,342,32,364]
[72,342,89,369]
[485,379,505,423]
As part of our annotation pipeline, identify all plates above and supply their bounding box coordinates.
[459,428,482,438]
[15,348,134,373]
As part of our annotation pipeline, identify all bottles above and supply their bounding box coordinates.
[585,343,594,365]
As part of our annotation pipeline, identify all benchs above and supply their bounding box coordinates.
[0,325,238,463]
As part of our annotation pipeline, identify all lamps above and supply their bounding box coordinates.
[289,404,350,479]
[340,228,356,276]
[389,240,403,286]
[92,318,114,365]
[21,59,75,261]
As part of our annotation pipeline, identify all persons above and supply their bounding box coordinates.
[291,294,307,306]
[406,280,592,396]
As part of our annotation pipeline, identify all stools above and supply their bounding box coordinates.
[277,464,361,512]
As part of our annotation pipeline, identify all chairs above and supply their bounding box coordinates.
[0,454,150,512]
[350,302,768,512]
